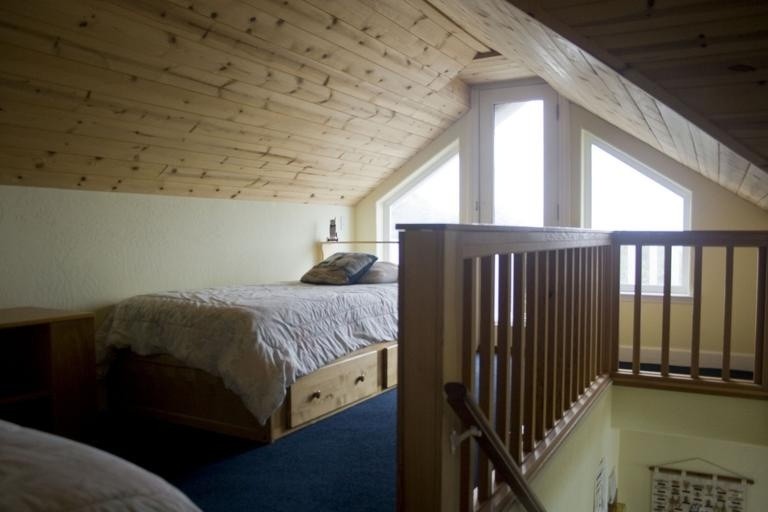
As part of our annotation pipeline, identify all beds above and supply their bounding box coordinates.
[105,280,399,445]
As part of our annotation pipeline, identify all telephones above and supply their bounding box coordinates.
[328,219,338,241]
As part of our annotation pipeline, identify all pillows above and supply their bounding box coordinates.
[301,250,398,285]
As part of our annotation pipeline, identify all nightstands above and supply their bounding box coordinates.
[1,305,102,440]
